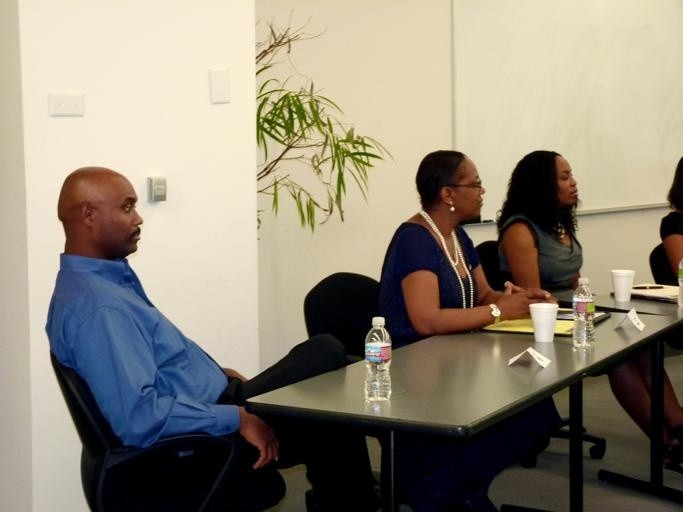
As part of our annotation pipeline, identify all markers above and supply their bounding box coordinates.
[485,219,493,222]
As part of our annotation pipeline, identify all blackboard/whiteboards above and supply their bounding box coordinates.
[451,3,683,225]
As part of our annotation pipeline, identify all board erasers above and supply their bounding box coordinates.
[460,218,479,223]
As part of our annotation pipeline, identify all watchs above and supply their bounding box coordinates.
[489,304,501,324]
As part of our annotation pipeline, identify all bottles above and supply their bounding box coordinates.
[571,277,594,348]
[363,317,392,402]
[677,257,682,307]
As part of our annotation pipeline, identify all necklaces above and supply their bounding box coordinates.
[555,224,565,240]
[419,210,474,309]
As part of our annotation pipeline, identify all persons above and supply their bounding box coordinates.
[45,168,415,512]
[661,158,683,286]
[374,151,564,512]
[492,151,683,474]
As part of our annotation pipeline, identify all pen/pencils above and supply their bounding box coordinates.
[632,286,664,289]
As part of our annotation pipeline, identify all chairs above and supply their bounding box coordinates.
[476,239,605,460]
[48,351,286,511]
[300,271,416,511]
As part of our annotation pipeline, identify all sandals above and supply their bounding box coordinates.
[663,406,683,475]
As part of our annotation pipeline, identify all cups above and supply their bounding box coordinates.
[529,342,558,373]
[527,302,558,343]
[609,268,635,303]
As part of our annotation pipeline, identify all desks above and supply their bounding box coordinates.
[547,281,681,503]
[244,306,678,511]
